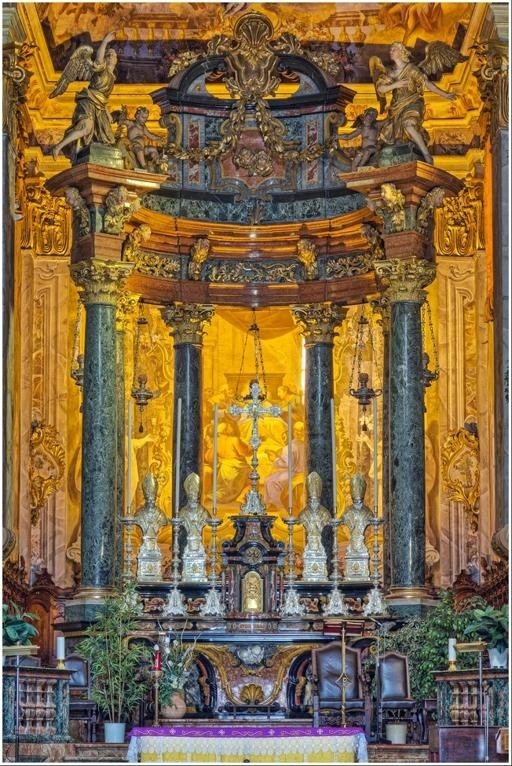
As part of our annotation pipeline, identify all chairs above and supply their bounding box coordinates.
[375,651,418,744]
[62,654,97,743]
[312,641,372,744]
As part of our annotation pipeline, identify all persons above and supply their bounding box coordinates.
[51,29,119,163]
[136,473,167,550]
[340,475,375,554]
[374,37,461,164]
[203,378,304,511]
[117,103,165,171]
[182,475,209,553]
[329,106,384,173]
[297,471,331,556]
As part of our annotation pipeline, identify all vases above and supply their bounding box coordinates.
[160,690,187,718]
[384,722,408,744]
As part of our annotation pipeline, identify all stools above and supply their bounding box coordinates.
[420,698,437,744]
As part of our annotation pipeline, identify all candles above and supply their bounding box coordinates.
[154,649,161,670]
[449,639,457,661]
[127,397,377,506]
[57,637,65,660]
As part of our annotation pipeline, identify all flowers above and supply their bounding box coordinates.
[159,617,202,710]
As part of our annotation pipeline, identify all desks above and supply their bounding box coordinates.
[125,727,368,763]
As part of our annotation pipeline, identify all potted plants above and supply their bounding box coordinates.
[74,576,154,743]
[464,602,509,669]
